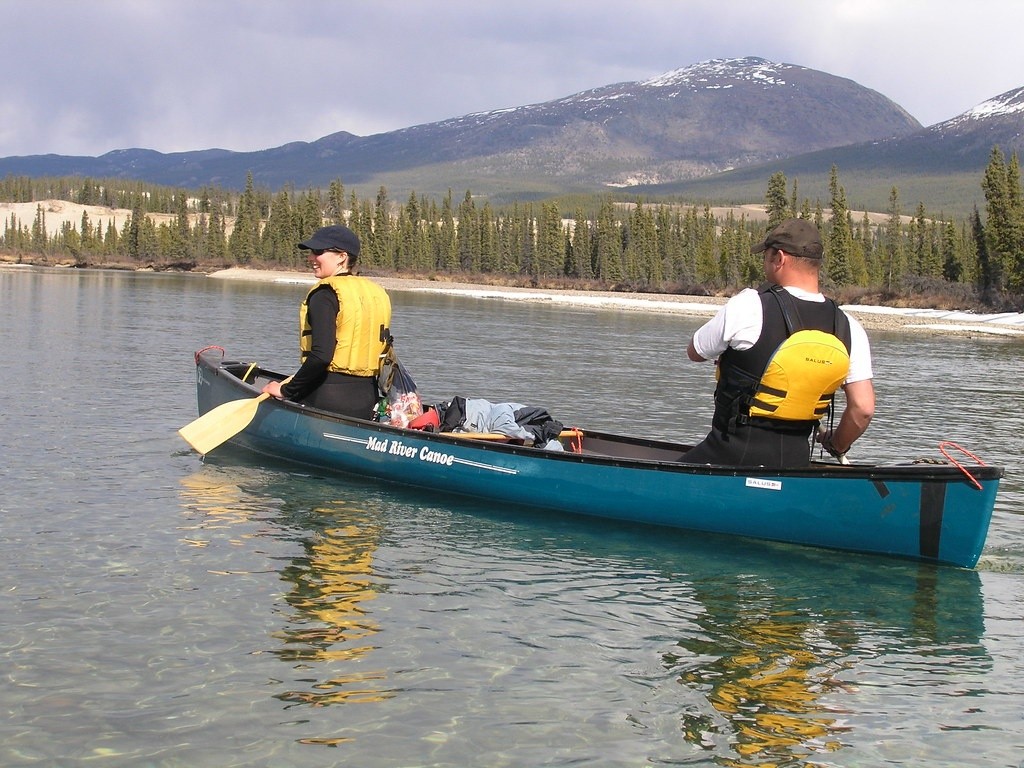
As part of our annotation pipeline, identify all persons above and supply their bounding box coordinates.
[675,217,875,468]
[262,225,392,419]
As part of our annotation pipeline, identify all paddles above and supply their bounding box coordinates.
[177,373,297,457]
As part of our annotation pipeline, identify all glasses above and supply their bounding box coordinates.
[309,248,336,256]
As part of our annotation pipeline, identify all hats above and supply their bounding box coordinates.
[750,218,823,259]
[297,225,360,257]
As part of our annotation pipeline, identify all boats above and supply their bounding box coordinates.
[190,347,1006,572]
